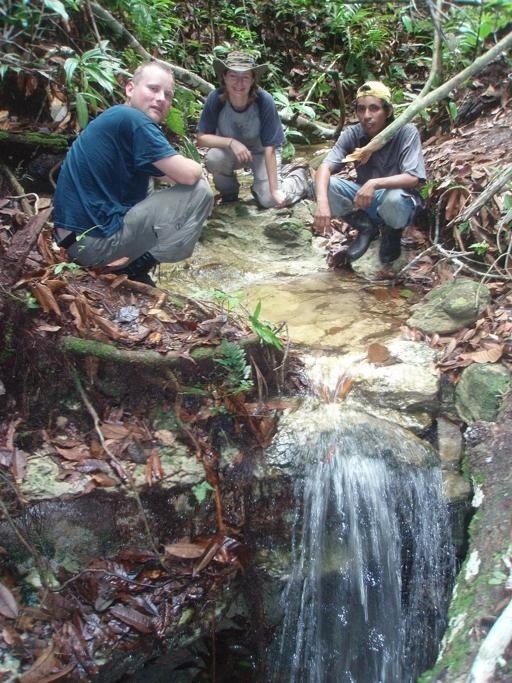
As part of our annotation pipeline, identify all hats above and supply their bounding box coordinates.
[355,81,392,104]
[213,51,269,83]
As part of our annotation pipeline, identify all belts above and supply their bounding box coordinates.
[57,232,76,249]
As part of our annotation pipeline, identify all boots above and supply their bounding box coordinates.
[111,251,160,288]
[379,223,402,263]
[340,209,380,260]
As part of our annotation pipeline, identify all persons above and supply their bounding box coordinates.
[52,62,213,288]
[198,52,314,209]
[312,80,427,262]
